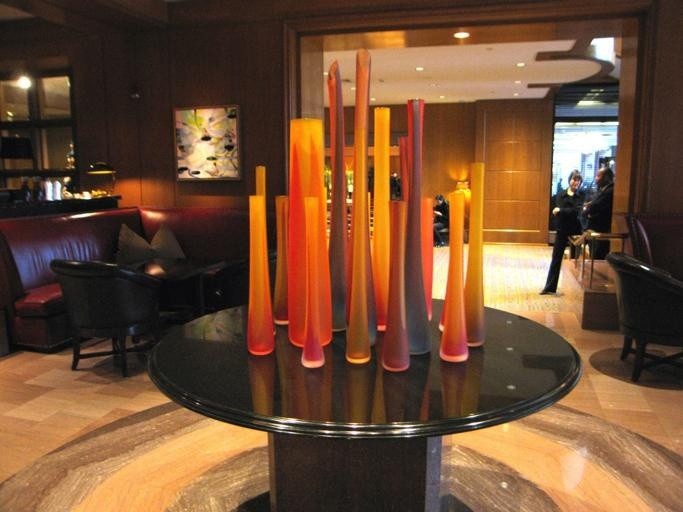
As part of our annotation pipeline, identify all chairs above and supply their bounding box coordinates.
[49,258,205,379]
[604,251,682,386]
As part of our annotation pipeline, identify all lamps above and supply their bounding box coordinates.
[85,159,118,199]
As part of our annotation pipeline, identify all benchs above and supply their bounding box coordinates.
[439,229,469,243]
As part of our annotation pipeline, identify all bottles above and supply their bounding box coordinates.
[43,181,61,201]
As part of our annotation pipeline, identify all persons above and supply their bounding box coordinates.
[580,164,617,257]
[538,170,589,295]
[429,194,449,247]
[389,170,402,201]
[556,177,562,192]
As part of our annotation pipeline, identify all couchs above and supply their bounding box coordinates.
[0,206,279,353]
[625,211,682,280]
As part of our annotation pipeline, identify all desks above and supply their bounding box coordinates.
[0,196,121,218]
[144,297,585,510]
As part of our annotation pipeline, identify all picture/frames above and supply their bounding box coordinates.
[171,103,243,182]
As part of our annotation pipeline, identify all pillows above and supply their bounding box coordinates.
[115,223,150,270]
[150,220,186,264]
